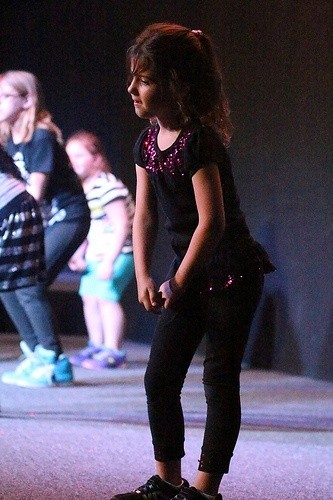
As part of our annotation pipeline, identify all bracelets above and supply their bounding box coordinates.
[169,278,186,295]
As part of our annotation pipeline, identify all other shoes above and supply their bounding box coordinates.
[68,342,128,371]
[1,340,72,388]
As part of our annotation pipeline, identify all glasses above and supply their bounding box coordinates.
[0,93,22,102]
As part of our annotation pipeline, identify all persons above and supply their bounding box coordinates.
[65,132,137,370]
[110,24,277,500]
[0,70,90,389]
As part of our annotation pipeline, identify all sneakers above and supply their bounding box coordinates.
[113,475,225,500]
